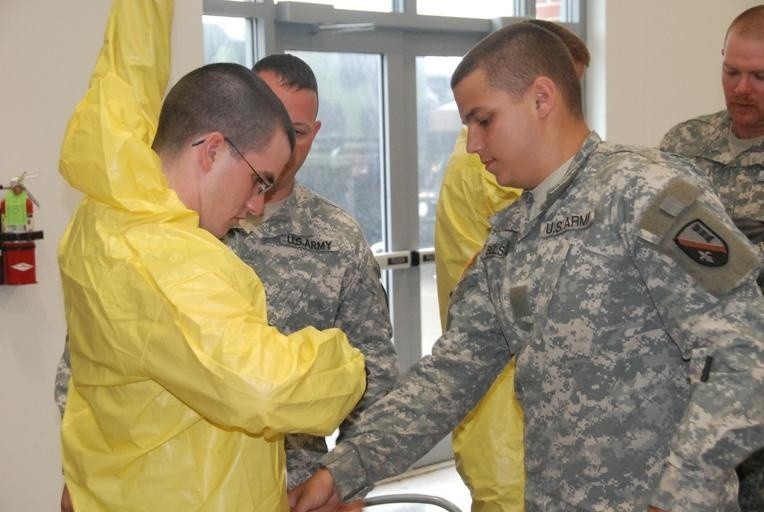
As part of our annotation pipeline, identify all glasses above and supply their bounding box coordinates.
[192,136,273,195]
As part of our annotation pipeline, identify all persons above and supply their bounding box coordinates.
[658,8,763,511]
[278,22,763,511]
[432,18,592,511]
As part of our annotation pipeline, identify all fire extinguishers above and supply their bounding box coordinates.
[0,171,44,285]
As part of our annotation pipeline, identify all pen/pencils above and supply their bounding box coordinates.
[701,356,713,382]
